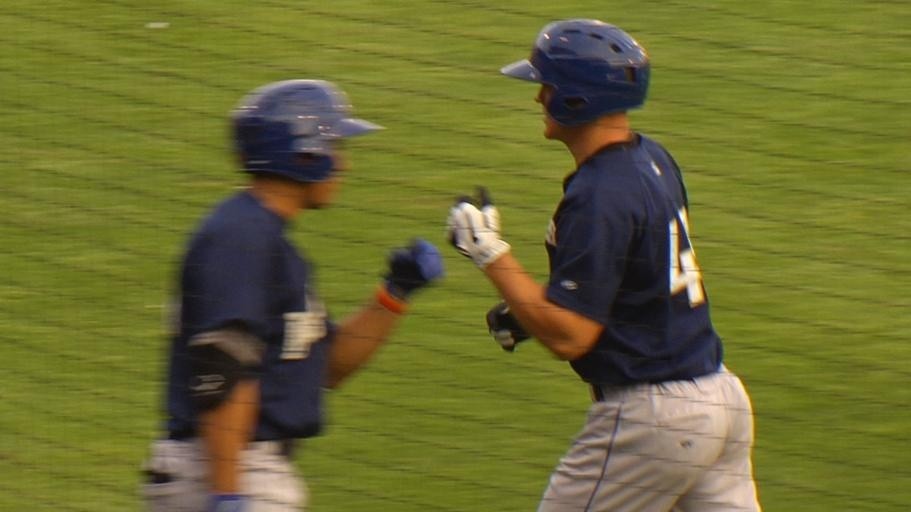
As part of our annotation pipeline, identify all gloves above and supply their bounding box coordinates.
[379,238,441,298]
[204,494,243,511]
[487,301,530,353]
[443,186,511,269]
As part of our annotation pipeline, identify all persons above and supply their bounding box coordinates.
[452,15,766,509]
[130,69,448,508]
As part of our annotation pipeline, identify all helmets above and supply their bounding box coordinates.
[498,15,650,124]
[229,72,385,182]
